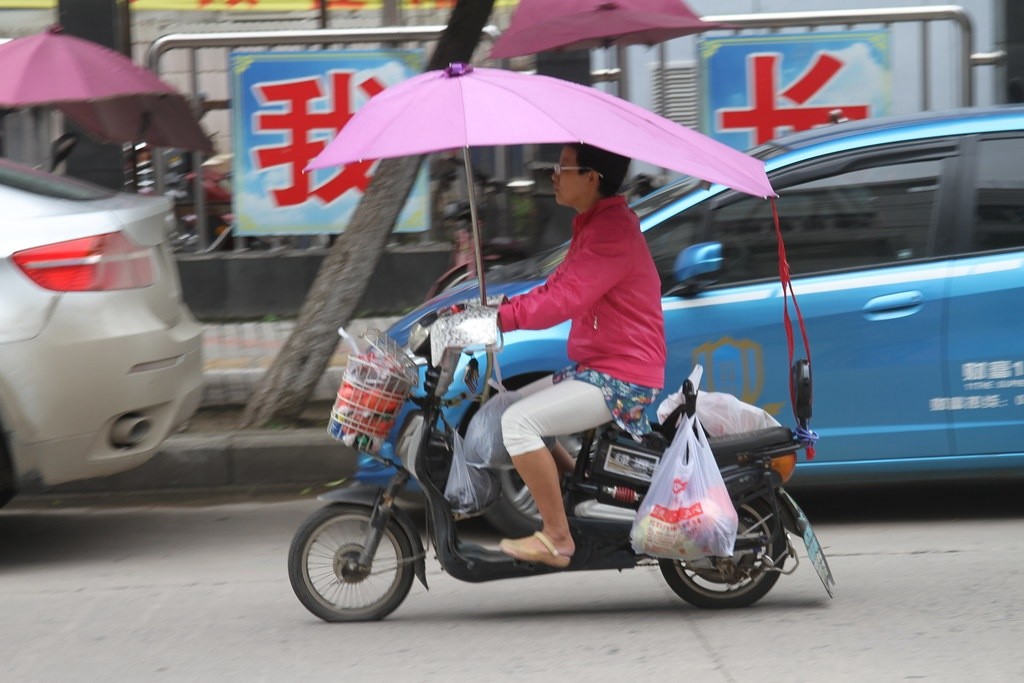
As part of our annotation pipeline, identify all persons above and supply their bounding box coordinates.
[64,132,124,189]
[533,43,622,254]
[497,141,664,569]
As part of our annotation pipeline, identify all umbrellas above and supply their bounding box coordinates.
[485,0,740,65]
[301,62,780,408]
[1,24,217,149]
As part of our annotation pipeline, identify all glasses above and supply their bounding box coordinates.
[553,162,603,178]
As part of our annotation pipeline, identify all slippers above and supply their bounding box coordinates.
[499,531,570,568]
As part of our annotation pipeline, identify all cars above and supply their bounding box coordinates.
[348,102,1023,538]
[0,154,207,519]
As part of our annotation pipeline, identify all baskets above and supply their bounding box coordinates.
[326,326,419,456]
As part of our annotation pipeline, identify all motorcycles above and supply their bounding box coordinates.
[287,294,837,624]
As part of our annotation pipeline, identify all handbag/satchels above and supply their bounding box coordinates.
[443,426,501,520]
[629,363,782,561]
[462,345,556,470]
